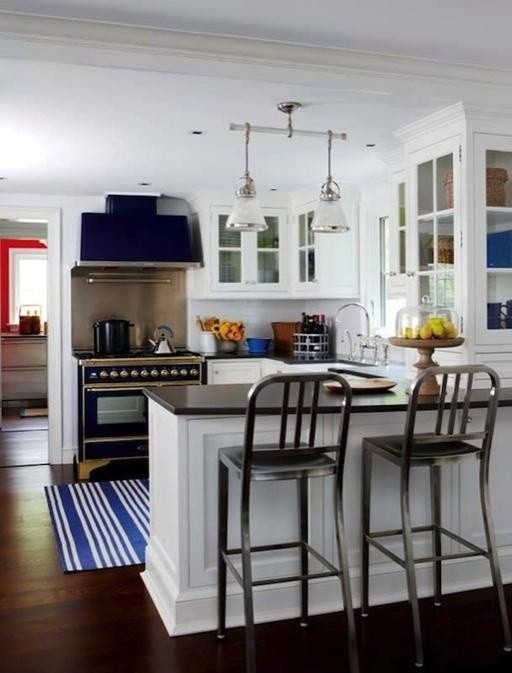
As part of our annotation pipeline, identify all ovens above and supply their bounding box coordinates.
[77,383,207,462]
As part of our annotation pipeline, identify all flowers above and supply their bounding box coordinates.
[192,312,247,343]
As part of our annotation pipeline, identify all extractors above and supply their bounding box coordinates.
[69,194,203,278]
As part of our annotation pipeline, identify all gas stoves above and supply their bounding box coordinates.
[75,350,204,360]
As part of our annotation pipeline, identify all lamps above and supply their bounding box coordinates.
[225,101,350,233]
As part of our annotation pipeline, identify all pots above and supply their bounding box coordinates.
[91,314,135,357]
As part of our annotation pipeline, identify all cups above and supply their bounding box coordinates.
[488,317,512,329]
[243,337,273,353]
[488,299,512,319]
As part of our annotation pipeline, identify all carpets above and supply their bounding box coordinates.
[43,476,165,574]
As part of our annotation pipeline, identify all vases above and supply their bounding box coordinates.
[216,339,238,353]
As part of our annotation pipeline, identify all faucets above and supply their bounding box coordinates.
[334,303,378,364]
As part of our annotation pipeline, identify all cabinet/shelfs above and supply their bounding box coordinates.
[0,331,49,412]
[292,181,359,301]
[186,189,292,302]
[464,101,511,353]
[386,170,407,285]
[393,101,463,353]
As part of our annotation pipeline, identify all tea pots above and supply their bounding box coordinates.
[147,325,176,356]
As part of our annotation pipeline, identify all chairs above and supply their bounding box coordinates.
[214,364,360,668]
[365,364,512,668]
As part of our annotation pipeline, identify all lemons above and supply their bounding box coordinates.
[402,317,459,340]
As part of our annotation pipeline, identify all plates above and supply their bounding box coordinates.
[324,377,398,394]
